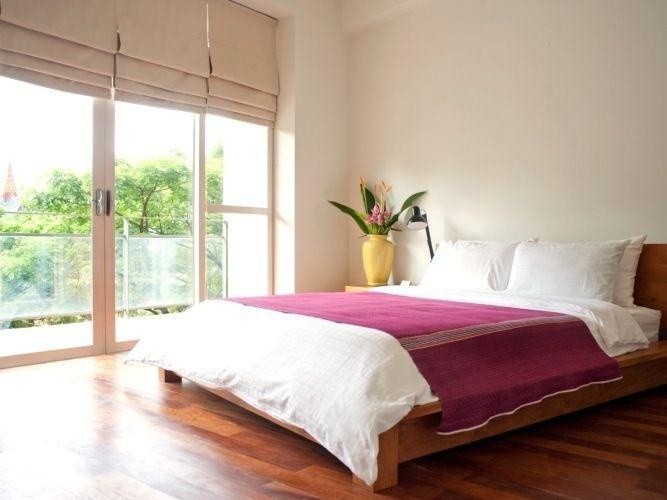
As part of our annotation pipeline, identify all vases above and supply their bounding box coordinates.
[361,233,395,286]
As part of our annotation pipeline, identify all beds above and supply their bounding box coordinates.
[161,243,667,494]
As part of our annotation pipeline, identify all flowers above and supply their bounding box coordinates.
[326,174,428,239]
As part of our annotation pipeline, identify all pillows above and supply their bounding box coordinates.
[420,233,651,307]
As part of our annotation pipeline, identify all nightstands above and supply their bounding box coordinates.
[344,283,380,292]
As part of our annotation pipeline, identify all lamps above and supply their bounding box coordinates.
[406,205,435,261]
